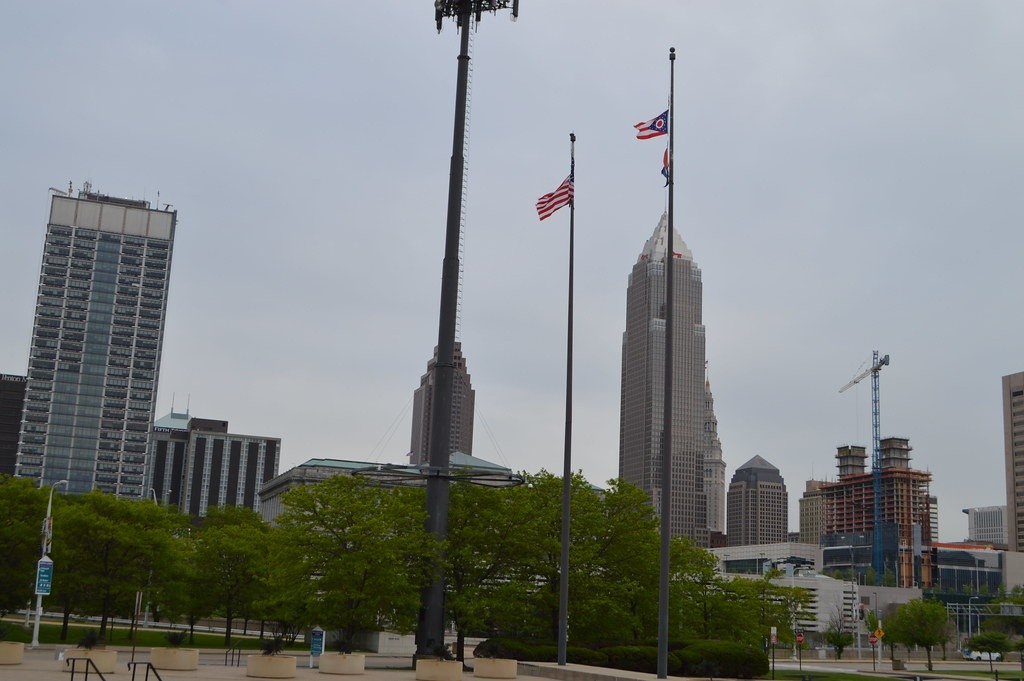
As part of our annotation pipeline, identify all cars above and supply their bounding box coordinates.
[963,647,1002,662]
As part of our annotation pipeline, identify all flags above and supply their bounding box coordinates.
[535,172,575,221]
[633,110,668,140]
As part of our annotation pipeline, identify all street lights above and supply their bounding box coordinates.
[27,479,68,650]
[969,597,979,638]
[872,592,878,629]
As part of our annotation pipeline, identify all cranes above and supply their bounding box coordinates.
[838,349,890,586]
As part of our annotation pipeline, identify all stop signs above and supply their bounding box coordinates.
[869,633,886,645]
[796,633,804,645]
